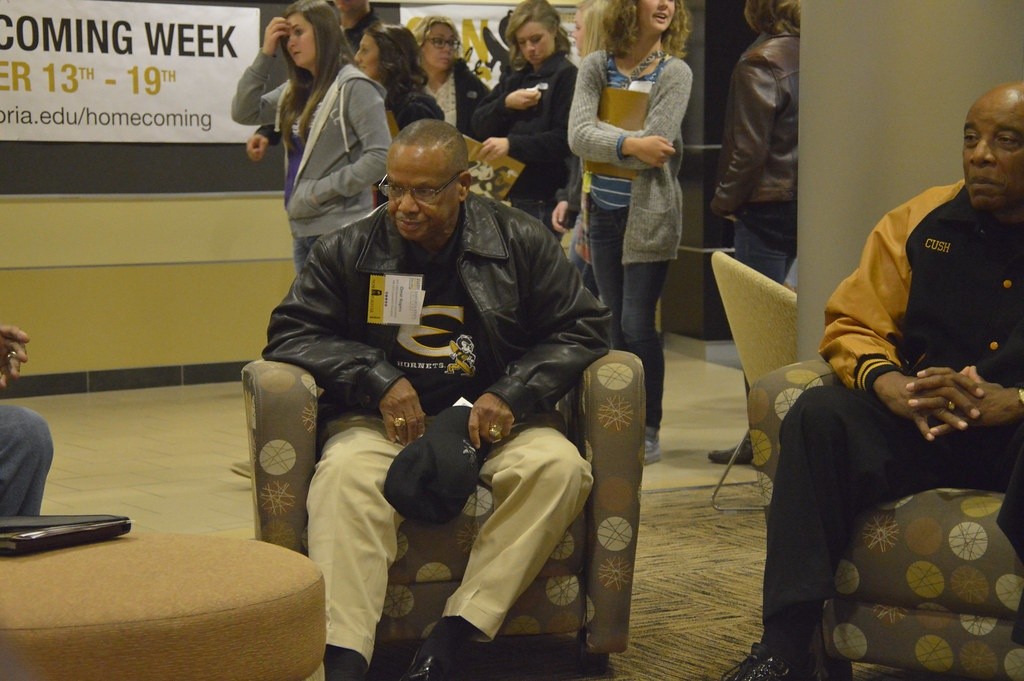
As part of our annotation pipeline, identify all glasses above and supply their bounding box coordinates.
[425,38,460,50]
[378,169,465,204]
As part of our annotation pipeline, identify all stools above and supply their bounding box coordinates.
[0,532,327,681]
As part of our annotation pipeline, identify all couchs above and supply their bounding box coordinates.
[743,356,1024,681]
[241,350,647,673]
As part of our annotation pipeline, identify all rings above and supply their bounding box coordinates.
[489,424,502,437]
[394,417,405,426]
[7,351,16,359]
[948,400,955,410]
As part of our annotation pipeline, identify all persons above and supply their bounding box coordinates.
[722,82,1024,681]
[231,0,692,478]
[708,0,799,465]
[261,121,614,681]
[0,325,54,516]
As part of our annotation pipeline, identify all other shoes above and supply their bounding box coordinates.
[721,642,809,681]
[230,460,251,480]
[708,434,754,465]
[643,439,662,466]
[399,646,450,681]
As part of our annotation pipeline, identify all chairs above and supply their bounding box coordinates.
[708,248,799,512]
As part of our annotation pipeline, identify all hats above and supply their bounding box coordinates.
[384,406,492,528]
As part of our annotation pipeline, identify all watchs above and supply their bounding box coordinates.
[1016,386,1024,404]
